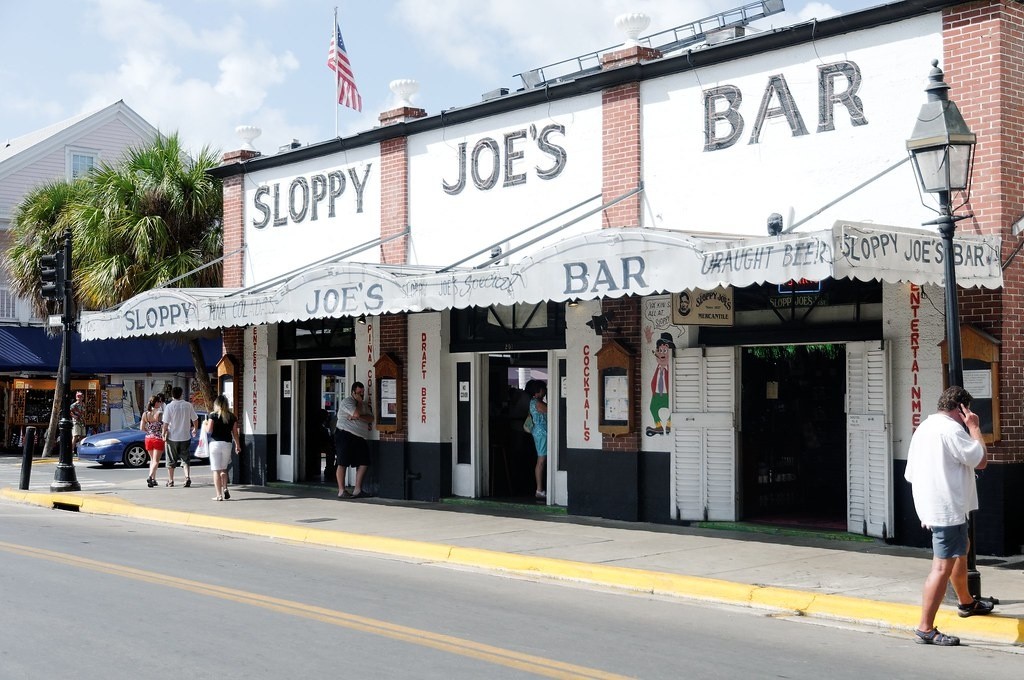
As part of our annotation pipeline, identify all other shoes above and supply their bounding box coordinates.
[152,478,159,486]
[536,490,547,499]
[147,476,154,488]
[166,481,174,487]
[183,477,191,487]
[224,489,230,499]
[213,496,222,501]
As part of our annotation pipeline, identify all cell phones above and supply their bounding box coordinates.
[958,402,963,414]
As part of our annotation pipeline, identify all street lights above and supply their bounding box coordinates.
[905,58,1001,609]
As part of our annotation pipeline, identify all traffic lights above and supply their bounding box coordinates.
[40,251,65,301]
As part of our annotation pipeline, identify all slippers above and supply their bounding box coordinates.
[337,490,354,499]
[353,490,370,498]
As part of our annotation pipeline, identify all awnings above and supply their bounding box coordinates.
[80,152,1002,341]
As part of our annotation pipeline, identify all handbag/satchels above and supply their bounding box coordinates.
[194,424,209,458]
[523,414,533,433]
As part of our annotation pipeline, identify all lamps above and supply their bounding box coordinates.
[568,299,578,307]
[357,315,367,324]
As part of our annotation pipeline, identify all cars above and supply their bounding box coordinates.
[76,409,211,468]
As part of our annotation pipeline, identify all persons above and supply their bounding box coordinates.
[162,387,198,487]
[677,292,691,317]
[333,380,374,499]
[70,391,88,455]
[139,396,166,487]
[157,393,167,410]
[205,395,243,501]
[901,382,994,646]
[523,377,548,497]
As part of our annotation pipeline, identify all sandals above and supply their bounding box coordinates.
[914,629,960,645]
[957,599,994,617]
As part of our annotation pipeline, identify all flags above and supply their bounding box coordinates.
[327,22,363,113]
